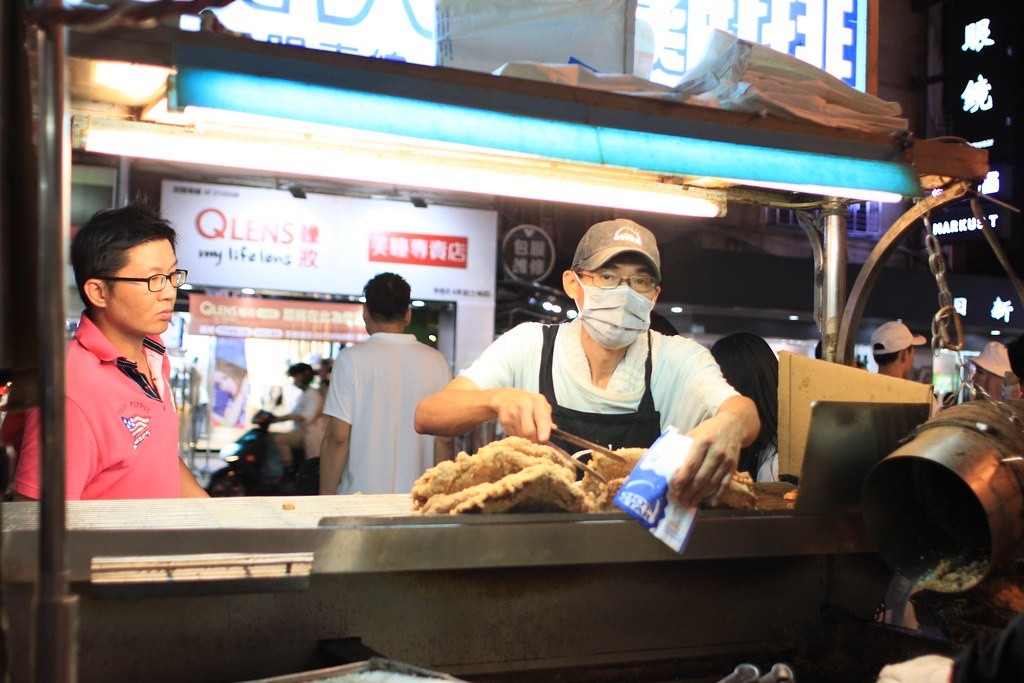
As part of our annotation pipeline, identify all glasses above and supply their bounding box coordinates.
[96,269,188,292]
[576,272,655,293]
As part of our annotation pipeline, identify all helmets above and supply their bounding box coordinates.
[288,363,314,385]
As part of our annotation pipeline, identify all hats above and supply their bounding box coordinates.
[969,341,1012,377]
[871,321,927,355]
[571,218,662,283]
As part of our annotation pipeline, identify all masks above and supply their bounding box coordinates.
[573,271,655,349]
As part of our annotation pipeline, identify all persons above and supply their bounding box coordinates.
[414,218,761,508]
[252,359,338,497]
[650,313,1024,482]
[319,271,455,496]
[0,197,214,500]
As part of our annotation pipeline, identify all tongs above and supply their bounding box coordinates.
[542,427,627,485]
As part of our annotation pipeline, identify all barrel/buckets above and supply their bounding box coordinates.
[855,399,1023,593]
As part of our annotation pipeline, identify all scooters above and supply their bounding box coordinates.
[201,407,314,499]
